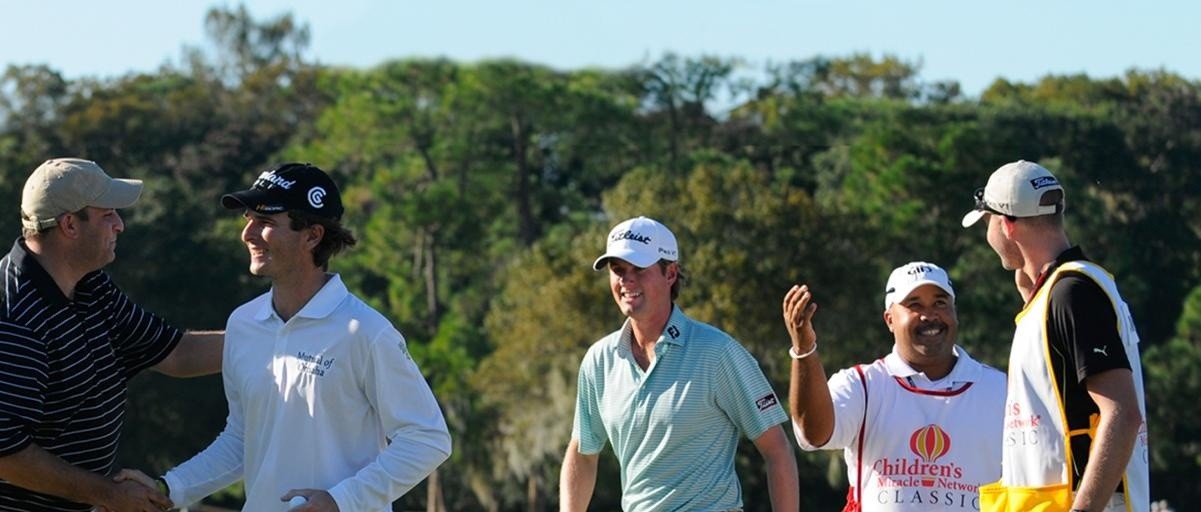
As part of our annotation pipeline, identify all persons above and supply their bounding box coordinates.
[0,158,226,512]
[100,161,454,512]
[782,261,1007,512]
[961,160,1151,512]
[559,215,799,512]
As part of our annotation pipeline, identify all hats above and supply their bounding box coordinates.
[220,164,344,220]
[592,216,679,271]
[961,159,1066,228]
[885,261,955,310]
[20,158,144,231]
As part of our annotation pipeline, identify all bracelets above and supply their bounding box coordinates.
[156,480,166,496]
[787,343,818,360]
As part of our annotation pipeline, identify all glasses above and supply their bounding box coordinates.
[973,187,1016,221]
